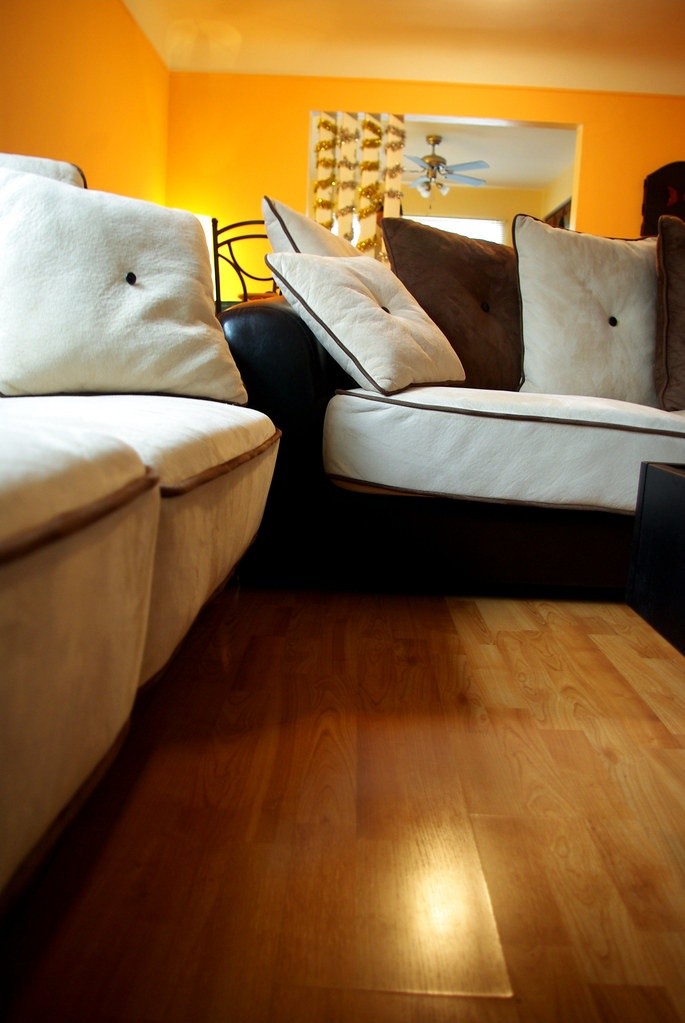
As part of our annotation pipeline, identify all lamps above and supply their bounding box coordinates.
[416,167,451,197]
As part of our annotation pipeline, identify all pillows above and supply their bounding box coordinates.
[263,253,466,395]
[379,217,522,392]
[655,215,685,413]
[512,214,662,412]
[0,152,86,189]
[262,194,364,256]
[0,166,249,406]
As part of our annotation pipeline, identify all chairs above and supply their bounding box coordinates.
[211,216,282,315]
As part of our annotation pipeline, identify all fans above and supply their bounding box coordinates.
[404,133,491,190]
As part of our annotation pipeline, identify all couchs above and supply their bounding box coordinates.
[218,295,685,603]
[0,392,282,920]
